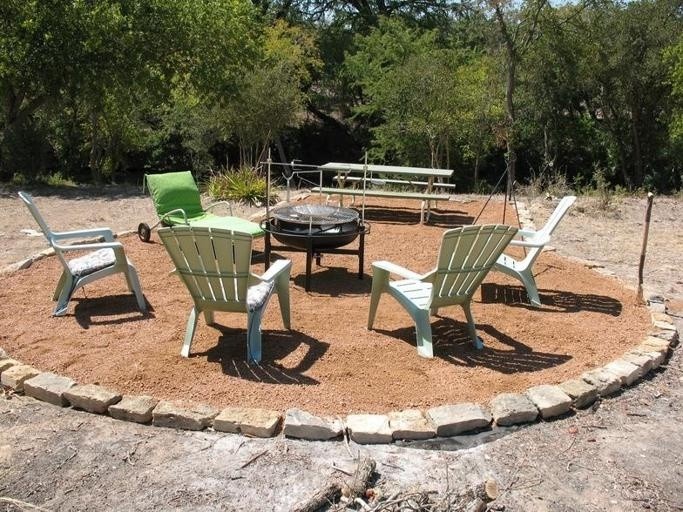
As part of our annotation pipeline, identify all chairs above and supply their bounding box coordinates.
[137,168,291,364]
[367,194,578,360]
[17,190,148,316]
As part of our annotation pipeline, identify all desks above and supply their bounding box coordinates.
[316,161,455,218]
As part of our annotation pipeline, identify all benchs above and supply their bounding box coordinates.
[309,177,457,226]
